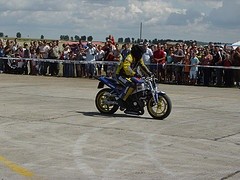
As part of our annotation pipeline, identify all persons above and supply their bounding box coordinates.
[0,33,240,90]
[113,44,157,108]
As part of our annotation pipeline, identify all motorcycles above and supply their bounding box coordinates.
[95,69,172,120]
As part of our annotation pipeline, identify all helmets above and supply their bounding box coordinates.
[131,45,146,61]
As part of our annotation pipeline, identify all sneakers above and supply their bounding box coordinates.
[116,98,130,109]
